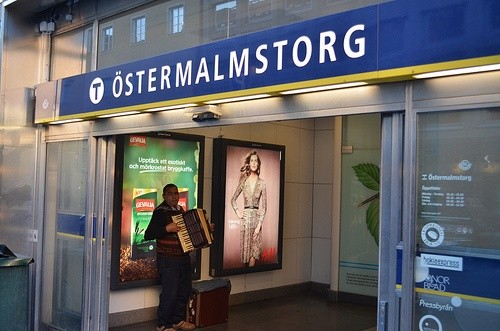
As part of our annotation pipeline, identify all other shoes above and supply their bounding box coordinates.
[173,321,195,330]
[156,326,175,331]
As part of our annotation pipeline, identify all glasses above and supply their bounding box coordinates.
[165,192,180,196]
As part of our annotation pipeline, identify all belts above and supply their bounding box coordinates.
[243,206,259,209]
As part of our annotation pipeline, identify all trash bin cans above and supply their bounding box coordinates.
[0,242,35,331]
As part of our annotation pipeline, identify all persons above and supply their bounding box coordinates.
[231,150,268,268]
[144,184,209,331]
